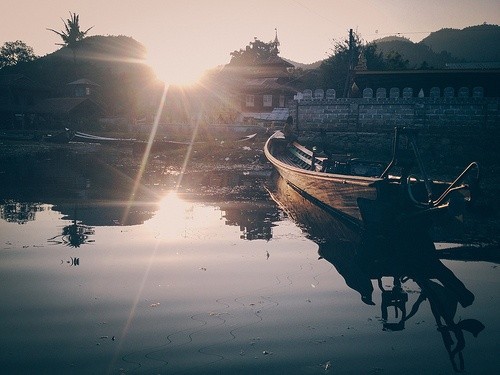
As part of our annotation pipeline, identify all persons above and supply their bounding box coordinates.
[283,115,298,149]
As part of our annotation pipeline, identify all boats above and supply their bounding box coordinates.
[263,128,482,240]
[131,131,259,152]
